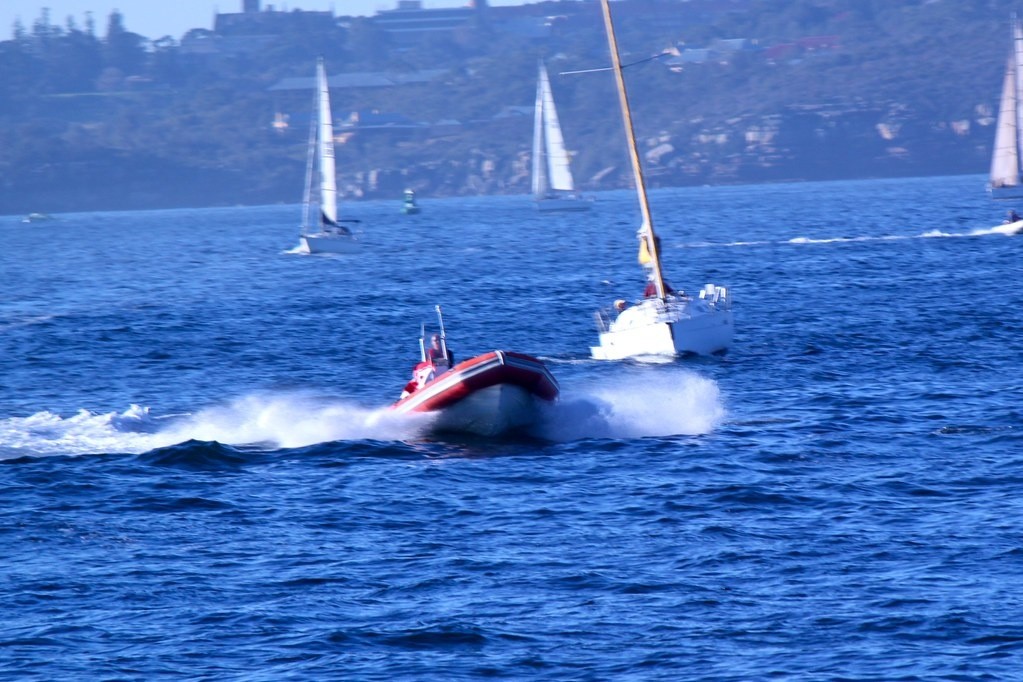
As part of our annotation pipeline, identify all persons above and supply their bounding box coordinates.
[423,333,455,372]
[642,272,677,299]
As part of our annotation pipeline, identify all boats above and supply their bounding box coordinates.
[383,301,561,438]
[398,188,421,216]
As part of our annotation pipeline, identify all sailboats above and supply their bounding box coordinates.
[987,5,1023,236]
[296,54,364,255]
[558,0,735,360]
[533,54,593,211]
